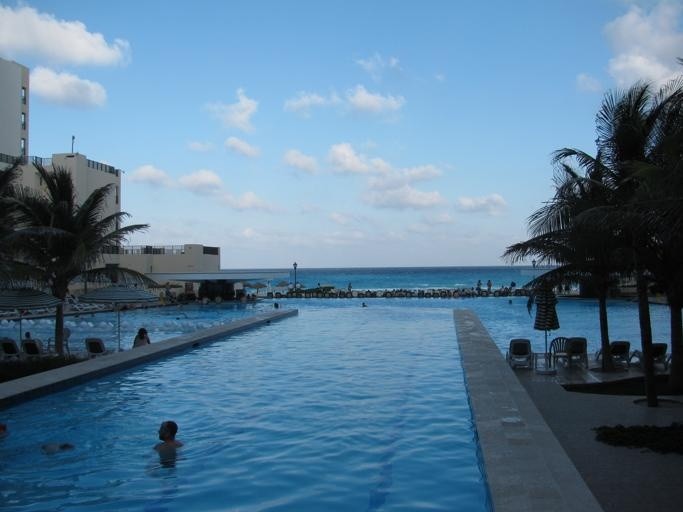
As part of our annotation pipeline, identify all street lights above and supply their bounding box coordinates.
[293,261,297,292]
[532,259,536,280]
[72,135,75,153]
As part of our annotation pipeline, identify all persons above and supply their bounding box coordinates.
[295,281,301,291]
[362,302,368,307]
[471,279,516,295]
[131,327,151,349]
[288,281,294,291]
[153,420,183,451]
[21,333,44,350]
[164,281,279,310]
[531,288,560,352]
[348,282,352,292]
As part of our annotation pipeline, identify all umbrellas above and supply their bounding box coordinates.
[79,285,157,349]
[0,286,65,341]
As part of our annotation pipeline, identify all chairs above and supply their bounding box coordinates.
[274,290,514,297]
[503,335,670,375]
[0,330,108,360]
